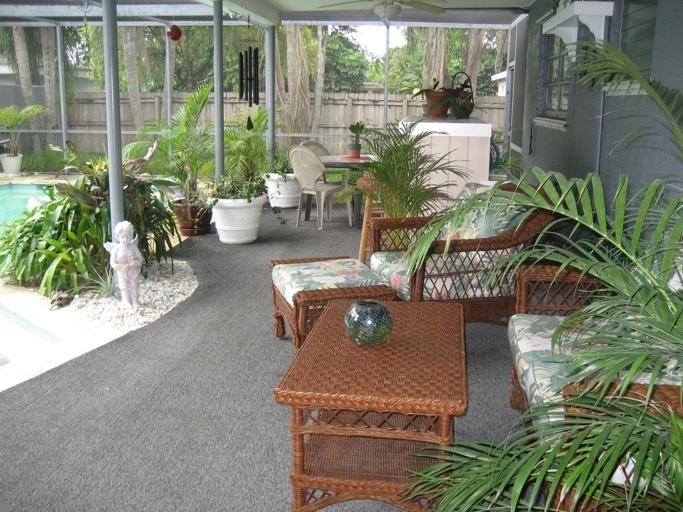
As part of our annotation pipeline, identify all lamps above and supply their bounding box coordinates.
[371,4,401,23]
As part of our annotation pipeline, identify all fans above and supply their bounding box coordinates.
[317,0,448,15]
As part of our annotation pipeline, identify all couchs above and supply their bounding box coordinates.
[506,245,682,511]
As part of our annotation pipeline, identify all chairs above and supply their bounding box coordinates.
[287,141,352,230]
[368,181,570,319]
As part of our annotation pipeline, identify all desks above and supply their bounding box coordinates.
[317,154,383,227]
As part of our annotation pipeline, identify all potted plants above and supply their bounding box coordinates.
[347,120,366,158]
[412,70,477,120]
[123,77,303,245]
[1,102,54,175]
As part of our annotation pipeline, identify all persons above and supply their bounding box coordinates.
[109,221,143,315]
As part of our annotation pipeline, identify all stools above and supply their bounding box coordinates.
[269,255,398,352]
